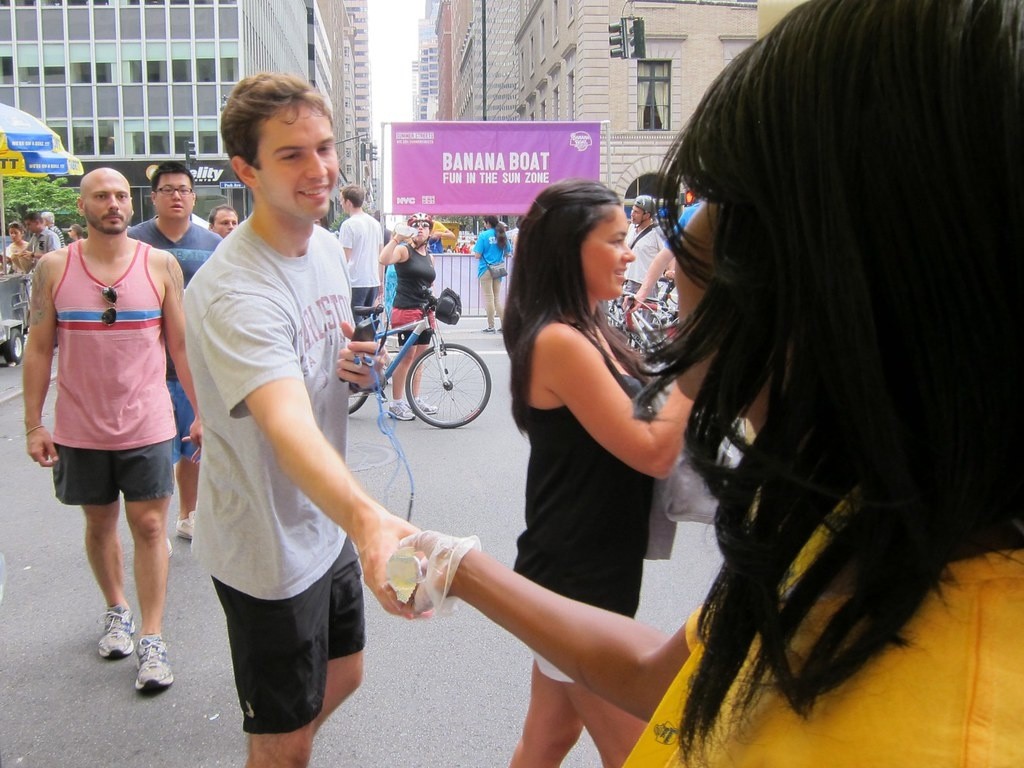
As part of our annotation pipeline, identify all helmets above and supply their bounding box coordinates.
[407,213,434,232]
[634,195,656,214]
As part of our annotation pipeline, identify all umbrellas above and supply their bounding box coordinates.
[0,101,62,274]
[0,149,84,177]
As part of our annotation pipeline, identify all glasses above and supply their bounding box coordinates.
[8,222,19,227]
[101,285,118,326]
[154,184,193,195]
[67,229,72,232]
[411,221,431,228]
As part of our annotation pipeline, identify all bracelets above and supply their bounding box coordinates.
[392,237,399,243]
[25,425,45,436]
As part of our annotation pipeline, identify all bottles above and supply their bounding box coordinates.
[395,224,418,238]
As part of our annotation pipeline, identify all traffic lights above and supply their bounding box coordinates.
[185,140,196,164]
[369,142,377,161]
[608,19,627,59]
[629,19,646,59]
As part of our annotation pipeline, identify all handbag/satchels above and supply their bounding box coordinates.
[487,263,508,279]
[664,419,746,526]
[643,456,678,558]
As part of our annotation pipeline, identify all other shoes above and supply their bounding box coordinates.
[482,328,495,334]
[52,346,59,356]
[497,328,503,332]
[176,510,194,540]
[167,539,173,558]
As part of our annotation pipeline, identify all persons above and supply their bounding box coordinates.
[623,200,703,312]
[508,180,695,768]
[393,0,1023,768]
[208,204,238,239]
[338,183,383,327]
[0,211,84,306]
[120,160,225,557]
[378,211,438,422]
[175,73,420,768]
[430,221,455,239]
[473,214,511,334]
[619,193,675,353]
[23,168,206,695]
[445,241,472,254]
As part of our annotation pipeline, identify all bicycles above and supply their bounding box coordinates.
[349,284,491,430]
[605,275,680,359]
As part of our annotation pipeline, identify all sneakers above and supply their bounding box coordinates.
[135,635,173,691]
[406,396,439,415]
[97,604,135,658]
[388,402,416,420]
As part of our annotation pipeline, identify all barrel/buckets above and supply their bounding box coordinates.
[429,237,443,254]
